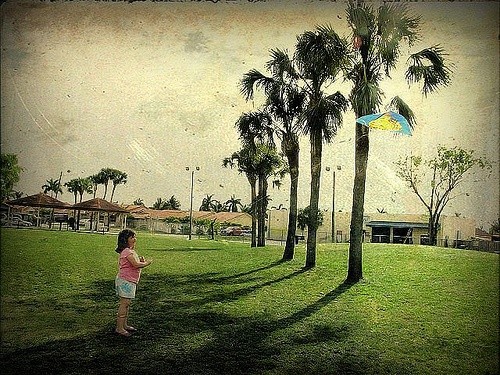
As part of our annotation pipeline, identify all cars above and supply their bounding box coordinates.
[1,217,33,227]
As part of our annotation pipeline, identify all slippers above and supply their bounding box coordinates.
[124,327,137,332]
[116,330,131,337]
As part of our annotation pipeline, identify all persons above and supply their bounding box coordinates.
[115,230,151,336]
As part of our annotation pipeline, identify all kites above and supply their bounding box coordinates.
[356,111,411,136]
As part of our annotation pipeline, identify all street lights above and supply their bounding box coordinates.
[185,166,200,240]
[325,166,341,242]
